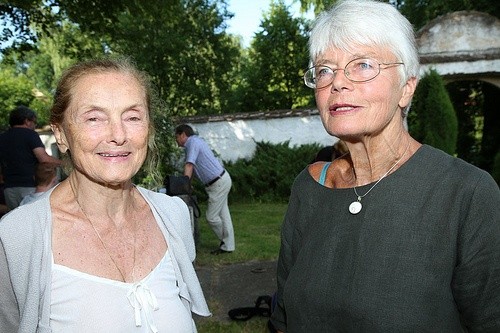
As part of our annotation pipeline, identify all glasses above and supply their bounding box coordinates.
[30,119,39,128]
[301,57,404,89]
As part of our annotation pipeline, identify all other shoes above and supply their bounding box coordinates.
[210,248,232,254]
[220,241,224,246]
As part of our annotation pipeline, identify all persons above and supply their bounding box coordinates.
[0,62,211,333]
[0,107,69,210]
[18,162,58,208]
[175,124,236,254]
[264,0,500,333]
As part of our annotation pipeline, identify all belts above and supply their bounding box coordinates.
[204,170,226,187]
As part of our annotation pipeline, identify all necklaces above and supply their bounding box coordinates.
[347,139,411,217]
[67,175,137,284]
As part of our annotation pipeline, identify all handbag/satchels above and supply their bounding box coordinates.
[163,175,192,196]
